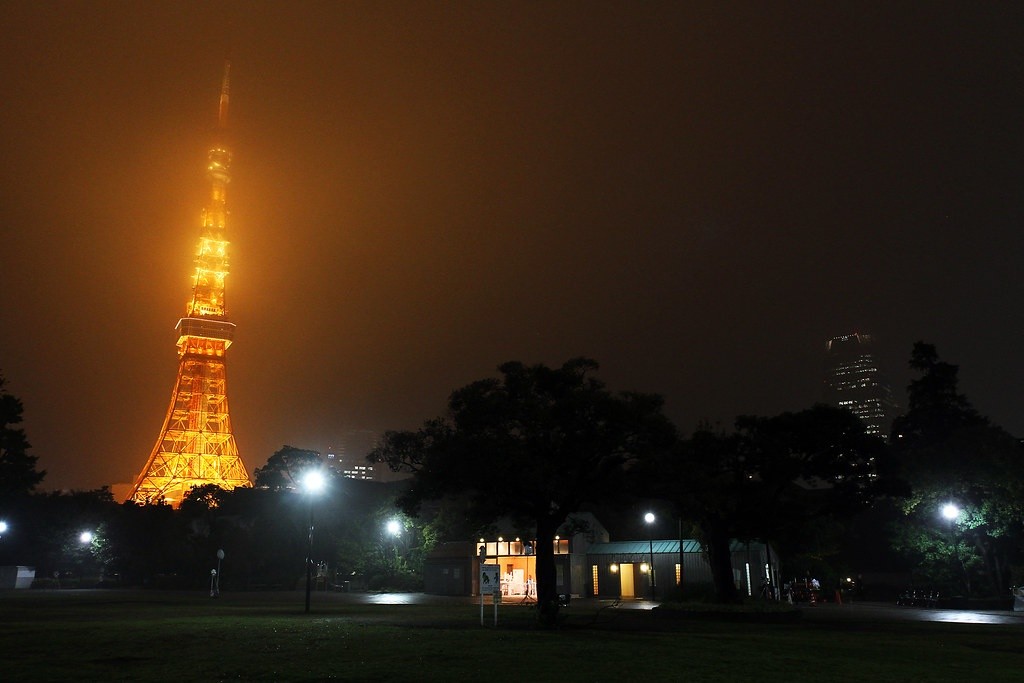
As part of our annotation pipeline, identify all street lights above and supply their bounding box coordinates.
[942,505,958,595]
[644,513,659,599]
[79,530,94,578]
[389,520,402,566]
[302,471,325,614]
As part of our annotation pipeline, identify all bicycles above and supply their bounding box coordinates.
[896,589,941,610]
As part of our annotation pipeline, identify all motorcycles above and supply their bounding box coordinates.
[757,577,822,609]
